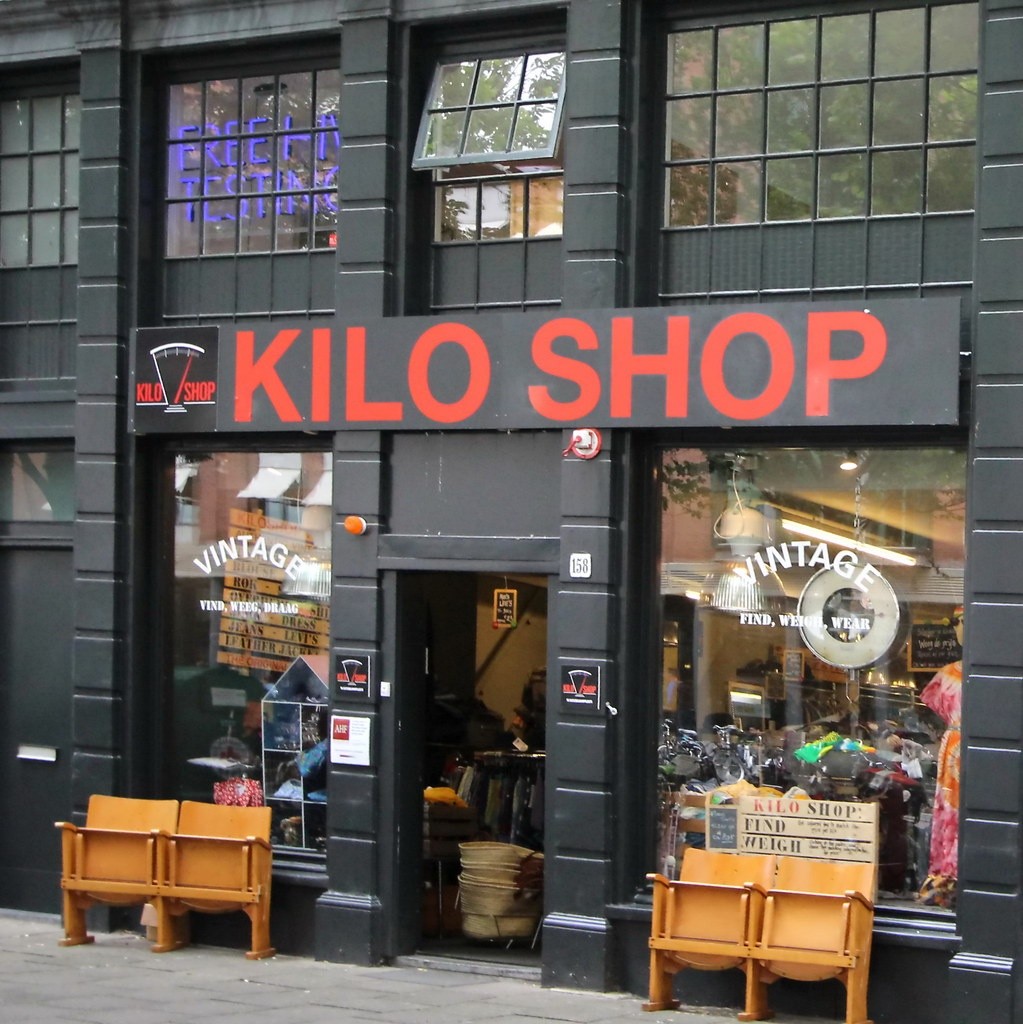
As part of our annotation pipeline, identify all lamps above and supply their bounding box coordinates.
[839,448,859,471]
[712,450,791,618]
[774,512,932,569]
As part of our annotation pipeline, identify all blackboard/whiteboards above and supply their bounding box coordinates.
[492,589,517,629]
[705,803,739,852]
[783,650,804,682]
[907,618,962,672]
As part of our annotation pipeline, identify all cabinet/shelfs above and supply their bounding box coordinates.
[262,654,329,860]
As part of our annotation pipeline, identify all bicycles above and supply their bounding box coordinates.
[659,717,939,900]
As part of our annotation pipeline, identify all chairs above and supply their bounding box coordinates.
[641,846,879,1024]
[52,794,277,961]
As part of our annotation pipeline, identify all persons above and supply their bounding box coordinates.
[851,720,934,893]
